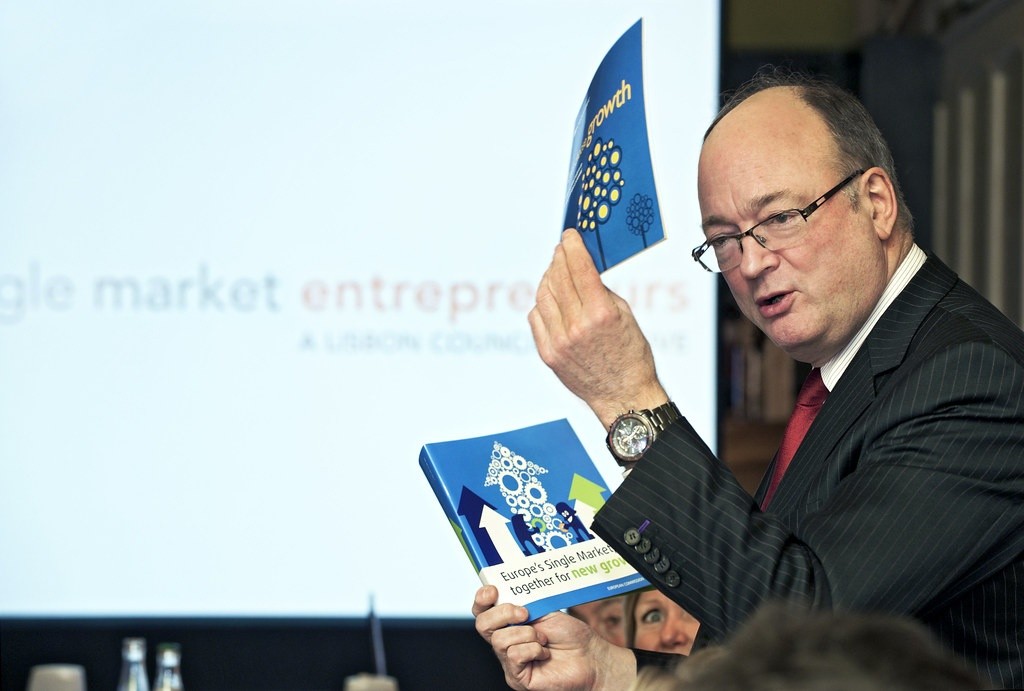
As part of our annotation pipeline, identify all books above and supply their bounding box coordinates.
[558,17,668,276]
[418,417,653,626]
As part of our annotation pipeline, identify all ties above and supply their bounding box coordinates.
[760,367,831,512]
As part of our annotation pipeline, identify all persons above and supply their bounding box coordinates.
[566,591,632,648]
[623,583,700,657]
[472,62,1024,691]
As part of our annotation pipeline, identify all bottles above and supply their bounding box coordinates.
[118,637,150,691]
[154,643,184,691]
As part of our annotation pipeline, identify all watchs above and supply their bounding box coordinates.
[605,401,682,466]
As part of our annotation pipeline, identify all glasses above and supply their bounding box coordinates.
[692,168,865,272]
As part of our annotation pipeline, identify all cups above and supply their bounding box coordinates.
[344,675,397,690]
[30,665,85,690]
